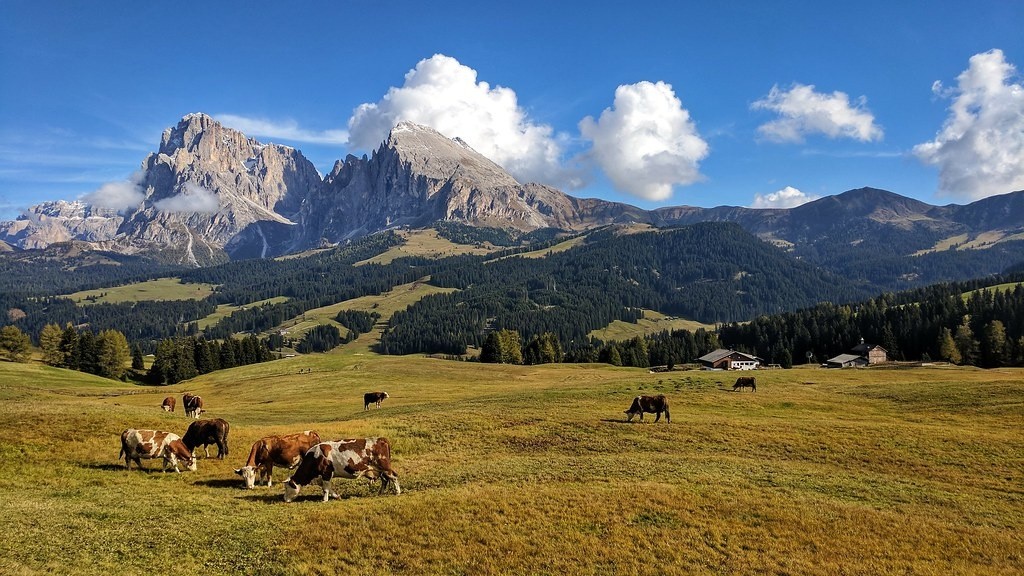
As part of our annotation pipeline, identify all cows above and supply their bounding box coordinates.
[183,393,205,418]
[281,437,401,503]
[161,397,176,412]
[731,377,757,393]
[182,418,229,460]
[363,391,390,410]
[119,428,197,474]
[624,394,670,424]
[232,431,321,490]
